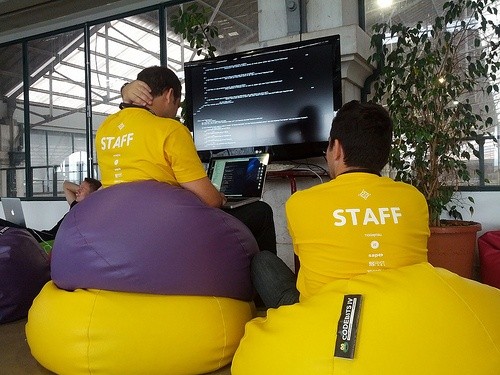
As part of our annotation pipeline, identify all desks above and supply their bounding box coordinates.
[266,170,324,274]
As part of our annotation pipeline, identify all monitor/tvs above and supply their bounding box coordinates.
[181,33,343,163]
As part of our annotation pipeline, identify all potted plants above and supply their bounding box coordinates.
[365,0,500,281]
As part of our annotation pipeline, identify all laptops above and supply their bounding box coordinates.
[206,152,270,210]
[1,198,26,228]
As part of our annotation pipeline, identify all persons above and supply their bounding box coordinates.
[0,178,102,243]
[94,66,277,308]
[249,99,431,310]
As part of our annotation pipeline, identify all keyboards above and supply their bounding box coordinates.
[267,164,295,171]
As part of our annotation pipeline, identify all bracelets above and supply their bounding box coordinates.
[120,83,129,99]
